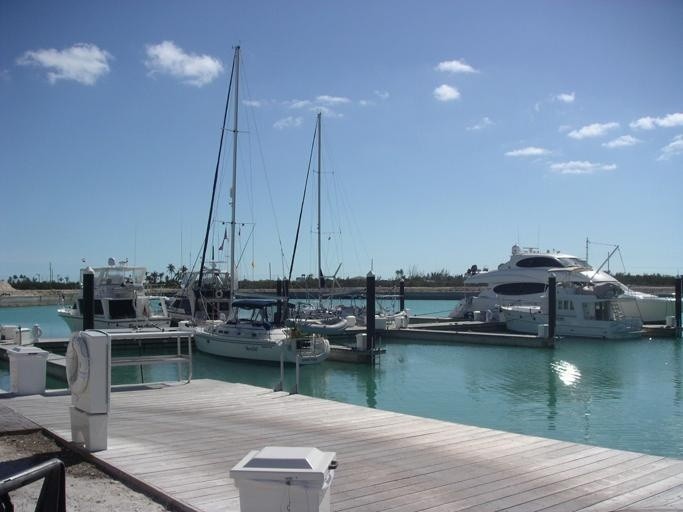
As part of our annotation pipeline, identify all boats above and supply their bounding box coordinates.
[448,236,683,339]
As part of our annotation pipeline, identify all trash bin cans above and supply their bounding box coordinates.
[356,333,368,351]
[7,346,49,395]
[230,446,338,512]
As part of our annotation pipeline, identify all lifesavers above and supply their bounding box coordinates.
[66,337,89,396]
[215,290,223,299]
